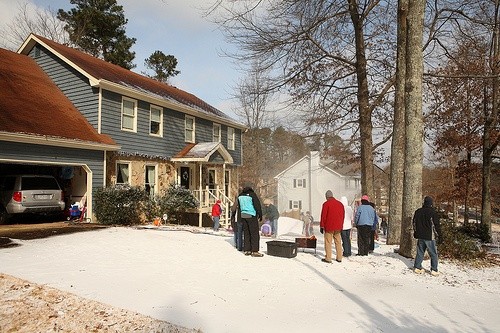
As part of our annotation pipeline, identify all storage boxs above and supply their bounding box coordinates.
[266,241,297,259]
[70,206,82,217]
[295,237,317,249]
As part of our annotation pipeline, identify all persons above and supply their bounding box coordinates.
[361,195,368,200]
[381,217,387,235]
[232,208,243,251]
[413,196,443,276]
[354,199,375,256]
[212,200,222,232]
[320,189,345,263]
[378,216,382,230]
[340,196,354,258]
[300,211,314,236]
[232,187,264,257]
[369,203,378,253]
[261,199,279,238]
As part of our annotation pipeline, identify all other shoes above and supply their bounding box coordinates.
[322,259,332,263]
[251,251,264,257]
[415,268,426,274]
[431,270,440,275]
[245,251,251,256]
[335,259,341,262]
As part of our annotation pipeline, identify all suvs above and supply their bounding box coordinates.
[0,173,66,225]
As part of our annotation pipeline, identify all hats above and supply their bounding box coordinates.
[361,195,368,201]
[217,200,221,203]
[263,199,270,205]
[424,196,432,205]
[326,190,333,198]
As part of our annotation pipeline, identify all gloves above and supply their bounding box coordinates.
[438,236,443,244]
[320,228,324,234]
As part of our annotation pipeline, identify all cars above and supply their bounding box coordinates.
[458,205,500,225]
